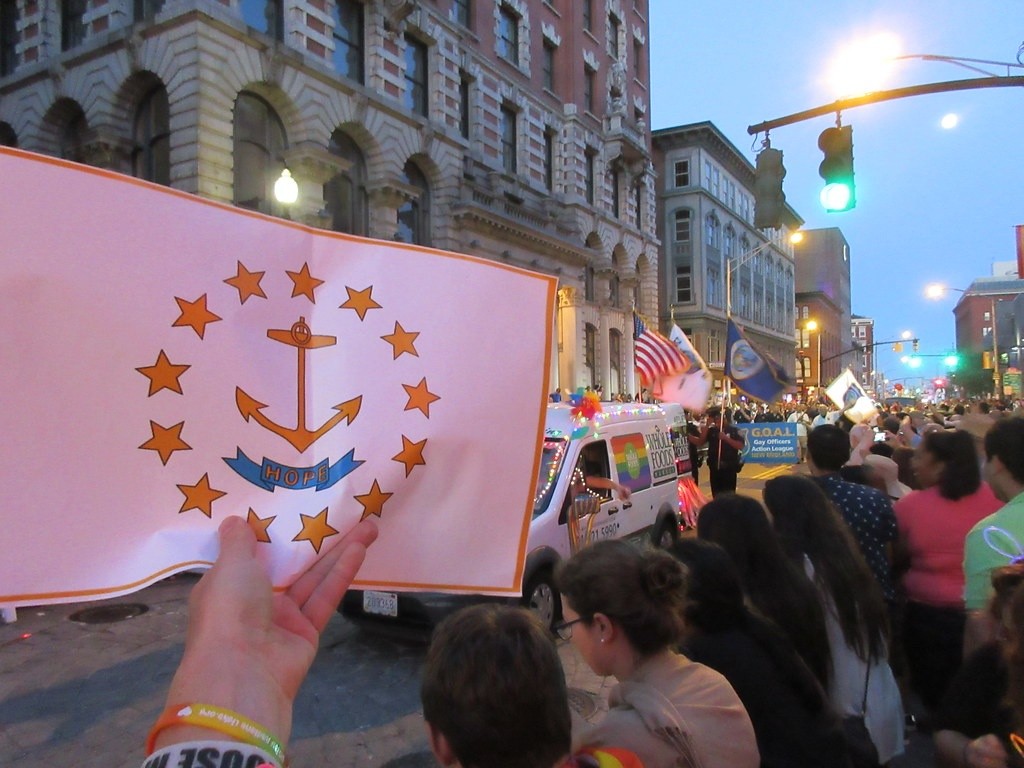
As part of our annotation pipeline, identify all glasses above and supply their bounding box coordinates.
[554,617,587,640]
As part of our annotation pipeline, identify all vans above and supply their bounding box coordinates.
[336,402,698,643]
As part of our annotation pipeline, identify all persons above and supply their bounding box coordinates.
[699,494,828,683]
[571,463,630,503]
[663,540,877,768]
[684,394,1024,512]
[554,539,761,768]
[992,564,1024,709]
[763,475,905,768]
[549,388,561,403]
[142,516,378,768]
[586,385,651,404]
[929,640,1024,768]
[419,603,644,768]
[962,416,1024,670]
[893,431,1008,733]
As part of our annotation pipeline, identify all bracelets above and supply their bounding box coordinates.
[141,742,279,768]
[144,703,286,768]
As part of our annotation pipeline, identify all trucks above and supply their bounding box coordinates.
[884,396,922,418]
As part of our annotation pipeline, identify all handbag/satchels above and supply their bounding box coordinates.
[843,714,879,768]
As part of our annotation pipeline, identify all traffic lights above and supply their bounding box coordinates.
[754,148,785,230]
[818,125,857,213]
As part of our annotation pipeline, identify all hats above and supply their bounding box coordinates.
[705,406,729,420]
[842,454,912,499]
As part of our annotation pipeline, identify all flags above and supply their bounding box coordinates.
[653,321,714,414]
[631,310,691,389]
[723,317,793,404]
[825,367,876,425]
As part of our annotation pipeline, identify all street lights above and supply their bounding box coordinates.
[727,232,804,404]
[927,285,1003,400]
[799,320,817,388]
[874,332,911,395]
[131,122,299,204]
